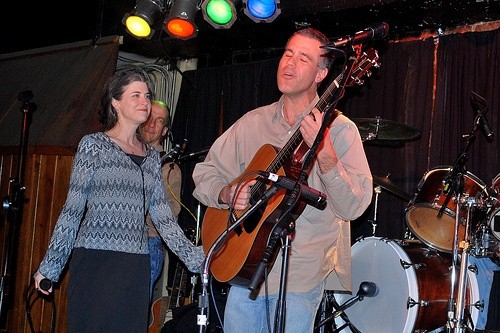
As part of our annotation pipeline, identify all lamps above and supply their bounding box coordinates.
[122,0,282,41]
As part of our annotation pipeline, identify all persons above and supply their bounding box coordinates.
[193,29,374,333]
[136,99,182,299]
[34,66,206,333]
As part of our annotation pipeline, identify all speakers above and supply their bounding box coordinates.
[161,296,227,333]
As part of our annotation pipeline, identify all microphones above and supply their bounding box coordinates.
[443,154,467,193]
[469,94,494,137]
[39,278,51,291]
[258,169,327,205]
[329,23,389,47]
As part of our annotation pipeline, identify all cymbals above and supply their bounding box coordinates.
[372,175,411,201]
[349,117,420,140]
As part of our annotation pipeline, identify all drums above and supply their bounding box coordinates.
[489,173,500,241]
[330,236,474,333]
[404,164,489,254]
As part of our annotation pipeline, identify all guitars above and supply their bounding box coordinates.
[200,48,382,288]
[147,225,197,333]
